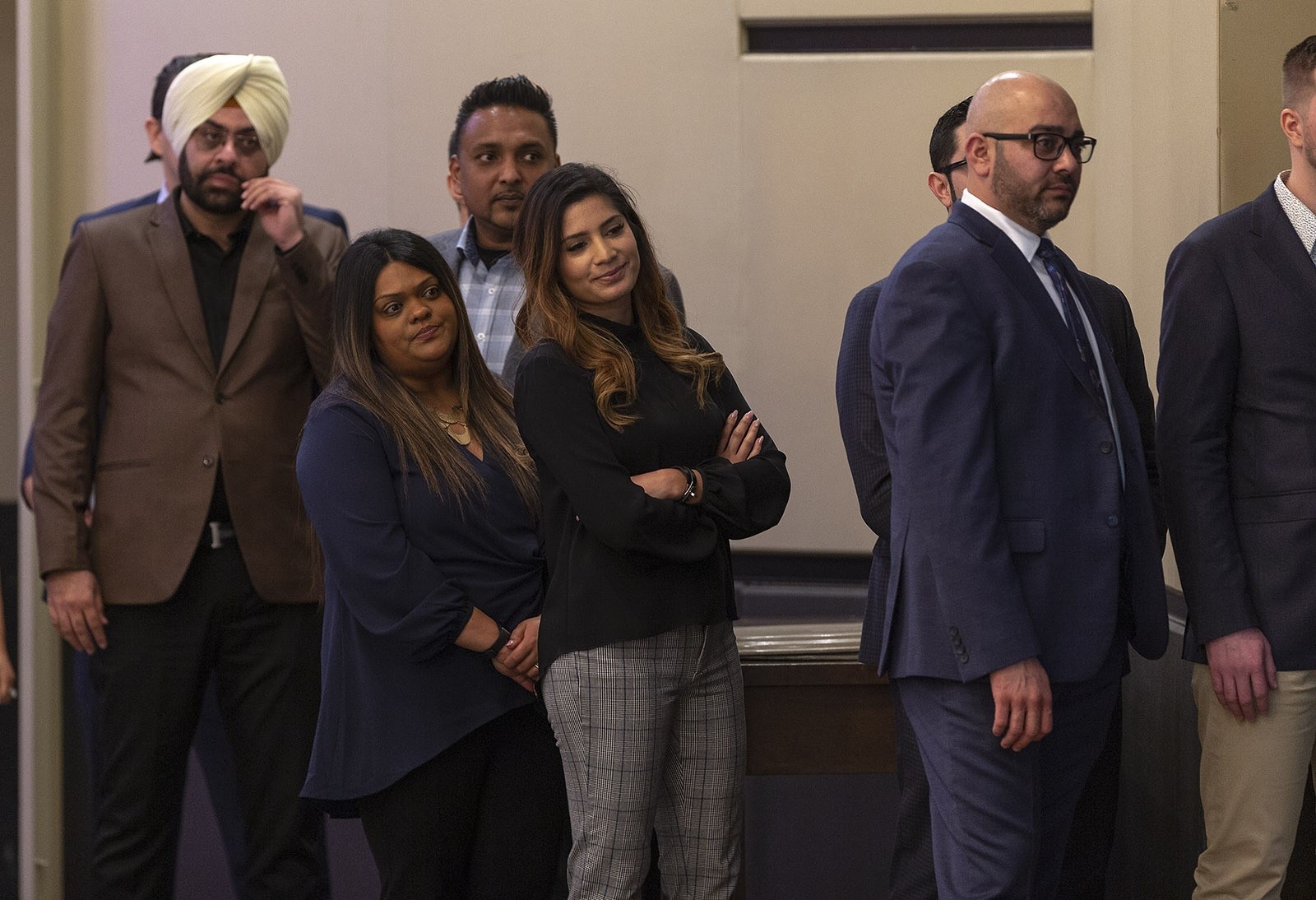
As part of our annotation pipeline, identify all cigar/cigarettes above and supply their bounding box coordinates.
[8,686,17,699]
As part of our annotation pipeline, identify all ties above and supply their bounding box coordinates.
[1037,240,1109,422]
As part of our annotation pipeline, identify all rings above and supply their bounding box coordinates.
[534,664,539,668]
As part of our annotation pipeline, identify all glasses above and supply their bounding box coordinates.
[193,128,261,155]
[982,132,1098,163]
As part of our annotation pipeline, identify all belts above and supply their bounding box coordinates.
[198,520,237,549]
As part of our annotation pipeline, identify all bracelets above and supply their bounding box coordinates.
[672,466,697,503]
[485,619,511,659]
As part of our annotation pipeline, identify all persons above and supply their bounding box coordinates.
[0,579,17,706]
[835,73,1171,900]
[423,74,686,392]
[21,55,353,900]
[295,229,562,900]
[513,163,792,900]
[1155,36,1316,900]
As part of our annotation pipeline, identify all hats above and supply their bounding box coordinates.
[161,53,291,168]
